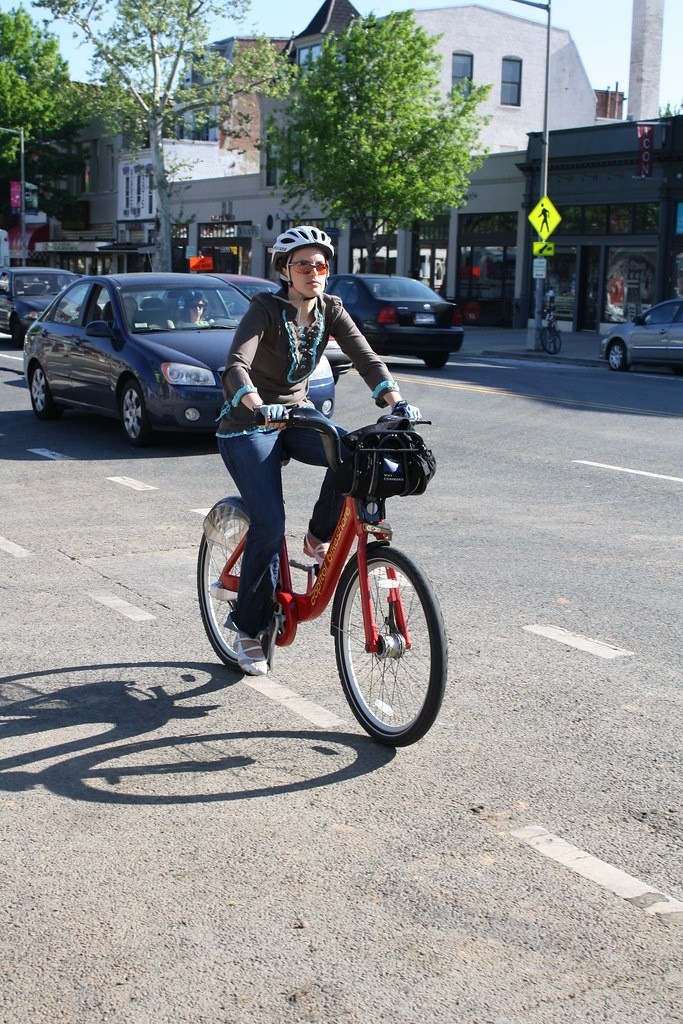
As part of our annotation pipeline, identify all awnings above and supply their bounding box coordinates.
[7,223,46,259]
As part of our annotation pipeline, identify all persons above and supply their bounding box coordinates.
[176,294,213,327]
[214,226,422,676]
[436,263,441,279]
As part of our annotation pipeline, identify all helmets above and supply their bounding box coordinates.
[271,226,334,272]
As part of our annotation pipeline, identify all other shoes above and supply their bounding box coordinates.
[233,632,267,675]
[303,532,330,569]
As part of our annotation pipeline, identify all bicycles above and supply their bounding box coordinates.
[193,405,450,749]
[536,295,563,355]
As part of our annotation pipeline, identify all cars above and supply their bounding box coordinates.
[0,265,82,350]
[322,273,465,369]
[598,296,683,376]
[20,272,355,449]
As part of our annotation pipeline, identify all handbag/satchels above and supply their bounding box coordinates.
[332,407,436,495]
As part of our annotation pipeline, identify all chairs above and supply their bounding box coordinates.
[139,298,170,326]
[100,302,114,328]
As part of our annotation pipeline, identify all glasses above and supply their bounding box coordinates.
[188,303,206,309]
[288,260,330,275]
